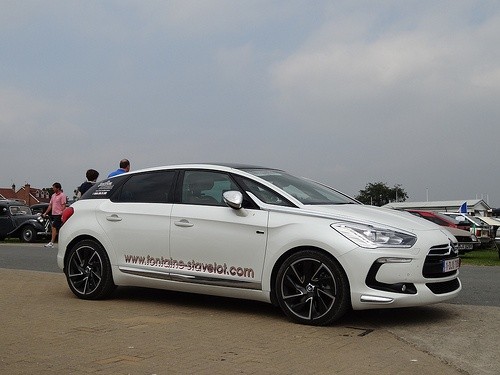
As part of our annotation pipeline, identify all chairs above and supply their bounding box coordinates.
[188,173,221,206]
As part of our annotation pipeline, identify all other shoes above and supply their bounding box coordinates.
[44,242,54,249]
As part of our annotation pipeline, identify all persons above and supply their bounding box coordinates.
[107,159,130,178]
[42,182,66,248]
[76,169,99,199]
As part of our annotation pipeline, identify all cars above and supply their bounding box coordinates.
[396,208,500,252]
[56,162,463,327]
[0,199,71,243]
